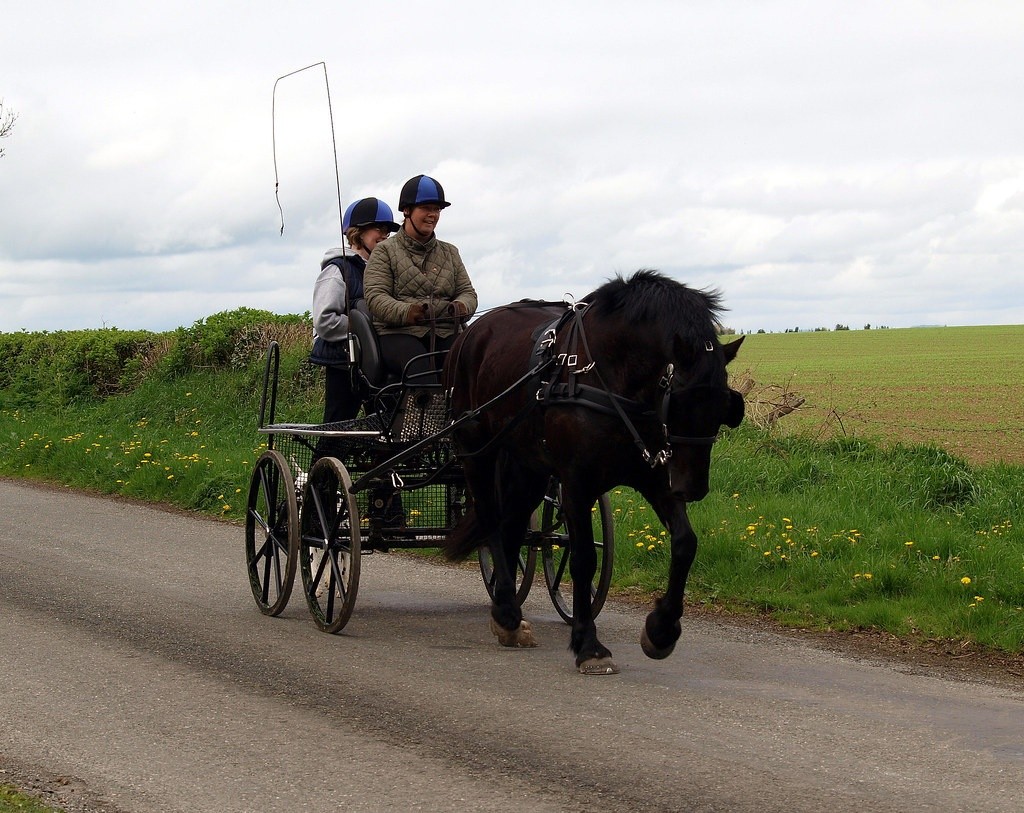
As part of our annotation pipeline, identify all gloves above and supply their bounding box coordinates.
[449,301,466,321]
[406,304,424,324]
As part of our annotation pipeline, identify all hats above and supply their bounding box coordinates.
[398,175,451,212]
[343,197,401,234]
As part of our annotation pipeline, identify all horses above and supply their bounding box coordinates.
[439,270,746,678]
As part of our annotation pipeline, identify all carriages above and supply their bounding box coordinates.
[244,269,745,676]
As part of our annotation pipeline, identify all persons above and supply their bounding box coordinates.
[300,197,415,539]
[363,175,478,385]
